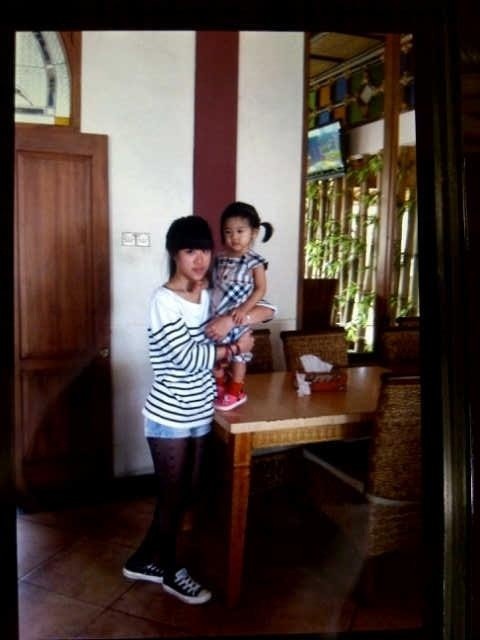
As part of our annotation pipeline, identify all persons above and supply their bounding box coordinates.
[192,202,274,410]
[122,217,275,606]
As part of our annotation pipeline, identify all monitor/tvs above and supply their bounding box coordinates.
[307,118,347,181]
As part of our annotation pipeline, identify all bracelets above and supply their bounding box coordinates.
[225,342,241,359]
[246,314,251,324]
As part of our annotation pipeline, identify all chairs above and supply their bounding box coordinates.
[381,325,421,367]
[292,370,423,631]
[246,328,275,372]
[280,326,348,371]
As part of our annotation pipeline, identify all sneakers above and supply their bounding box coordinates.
[162,568,212,604]
[217,372,232,397]
[122,556,164,583]
[214,393,247,410]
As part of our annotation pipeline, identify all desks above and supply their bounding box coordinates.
[212,367,381,605]
